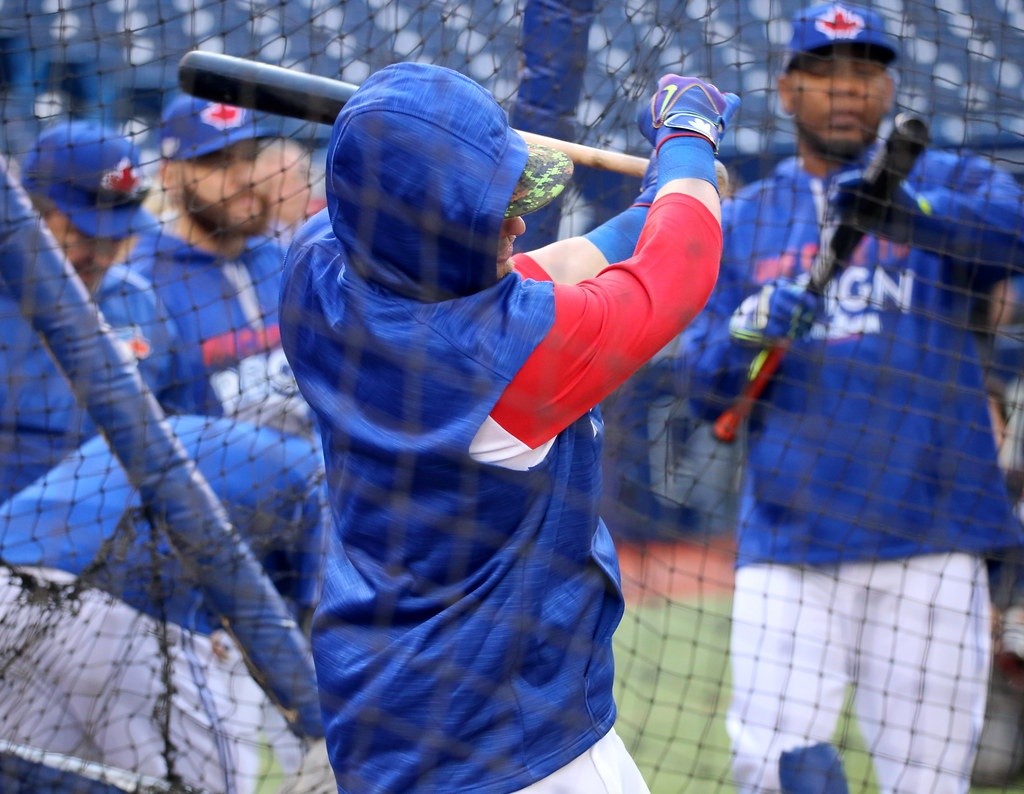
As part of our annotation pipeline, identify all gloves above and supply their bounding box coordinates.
[729,272,822,349]
[823,172,917,244]
[635,72,742,157]
[628,148,659,208]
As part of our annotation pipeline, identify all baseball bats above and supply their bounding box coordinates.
[178,50,729,200]
[711,112,931,445]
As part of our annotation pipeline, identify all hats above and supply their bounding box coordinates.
[505,144,576,218]
[784,1,900,74]
[18,119,160,239]
[156,93,291,161]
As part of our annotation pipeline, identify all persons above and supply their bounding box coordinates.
[0,122,161,503]
[684,3,1023,794]
[282,63,741,794]
[0,396,338,794]
[100,92,320,449]
